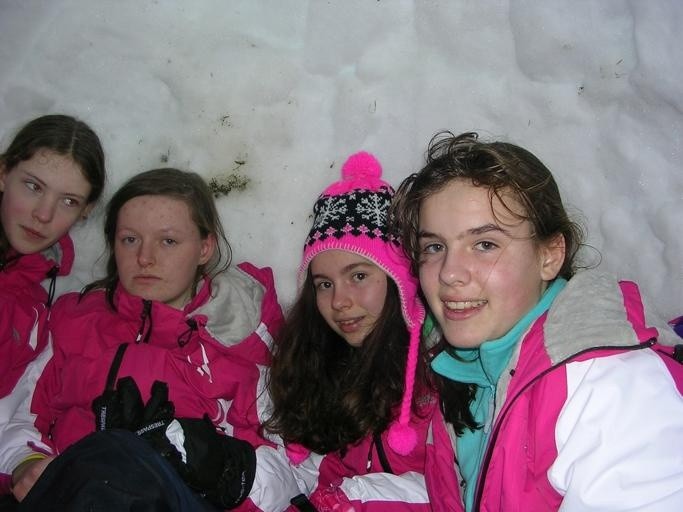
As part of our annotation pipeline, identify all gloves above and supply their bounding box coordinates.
[96,378,257,511]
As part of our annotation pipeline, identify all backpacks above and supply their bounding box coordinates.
[8,427,206,511]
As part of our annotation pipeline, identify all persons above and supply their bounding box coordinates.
[0,113,106,466]
[254,149,466,512]
[385,132,683,511]
[0,167,308,512]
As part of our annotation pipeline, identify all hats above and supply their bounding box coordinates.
[288,150,426,463]
